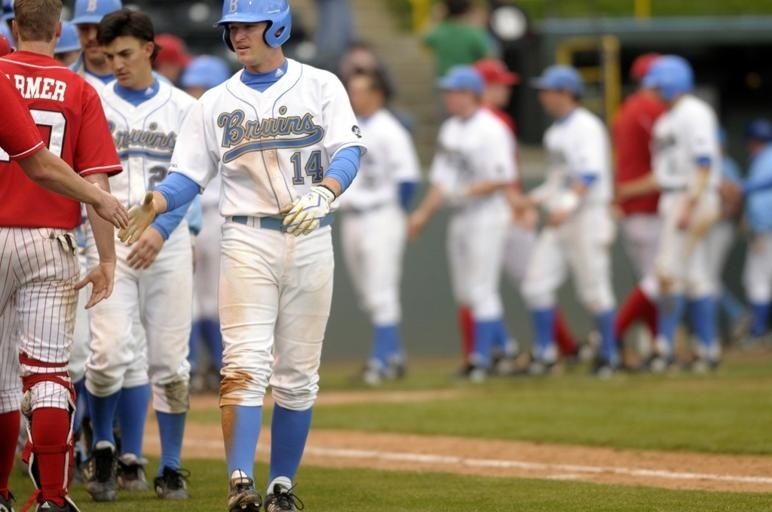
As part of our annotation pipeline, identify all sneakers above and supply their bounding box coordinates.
[36,496,82,512]
[75,437,306,512]
[1,491,16,512]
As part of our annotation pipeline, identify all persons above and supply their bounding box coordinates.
[0,0,368,512]
[424,0,556,146]
[337,44,772,389]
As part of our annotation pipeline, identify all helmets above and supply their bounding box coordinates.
[213,0,294,51]
[643,56,694,104]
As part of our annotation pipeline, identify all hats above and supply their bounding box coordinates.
[53,21,81,54]
[68,1,123,25]
[155,36,228,88]
[436,54,663,110]
[750,121,771,140]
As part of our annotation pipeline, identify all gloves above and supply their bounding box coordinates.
[118,191,157,247]
[279,188,335,237]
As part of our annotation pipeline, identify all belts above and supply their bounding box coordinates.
[233,213,335,232]
